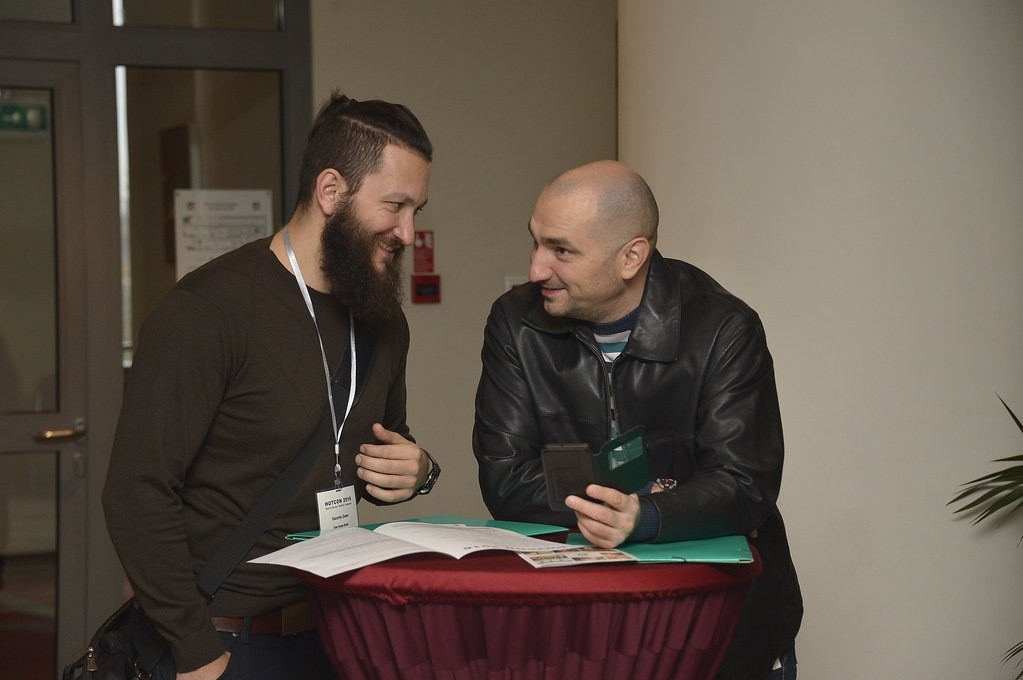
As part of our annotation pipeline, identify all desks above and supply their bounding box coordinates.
[290,527,764,680]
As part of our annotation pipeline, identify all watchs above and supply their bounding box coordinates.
[417,448,441,495]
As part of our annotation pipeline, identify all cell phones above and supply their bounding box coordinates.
[542,442,598,512]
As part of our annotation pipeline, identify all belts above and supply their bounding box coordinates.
[210,600,317,636]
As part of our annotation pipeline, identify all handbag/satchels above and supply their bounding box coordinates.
[61,595,170,680]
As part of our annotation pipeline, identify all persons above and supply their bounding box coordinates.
[99,91,432,680]
[472,160,804,680]
